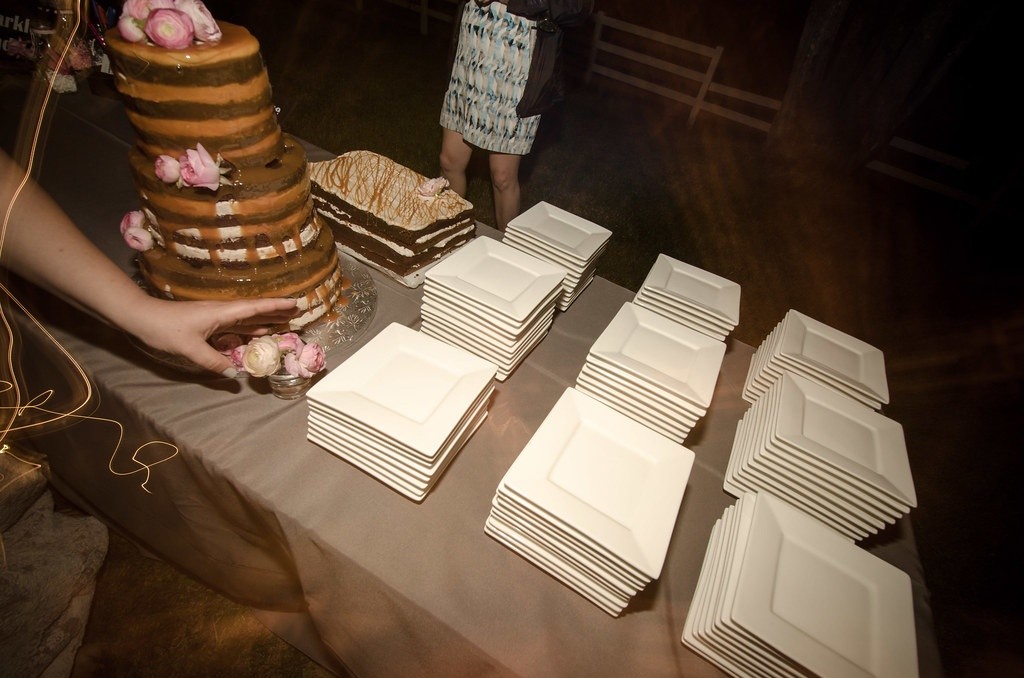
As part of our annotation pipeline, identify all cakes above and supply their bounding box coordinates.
[309,148,476,287]
[102,20,342,335]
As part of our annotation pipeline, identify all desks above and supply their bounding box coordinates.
[0,126,942,678]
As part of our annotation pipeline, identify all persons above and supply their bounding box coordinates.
[0,146,300,381]
[438,0,595,233]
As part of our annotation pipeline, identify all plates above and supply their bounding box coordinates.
[302,201,923,678]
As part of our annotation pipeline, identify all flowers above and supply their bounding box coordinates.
[120,211,154,252]
[117,0,223,51]
[153,141,233,191]
[45,41,93,94]
[219,326,327,378]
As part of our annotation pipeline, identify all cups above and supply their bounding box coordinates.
[269,365,311,400]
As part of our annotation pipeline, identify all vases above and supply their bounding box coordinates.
[267,374,312,400]
